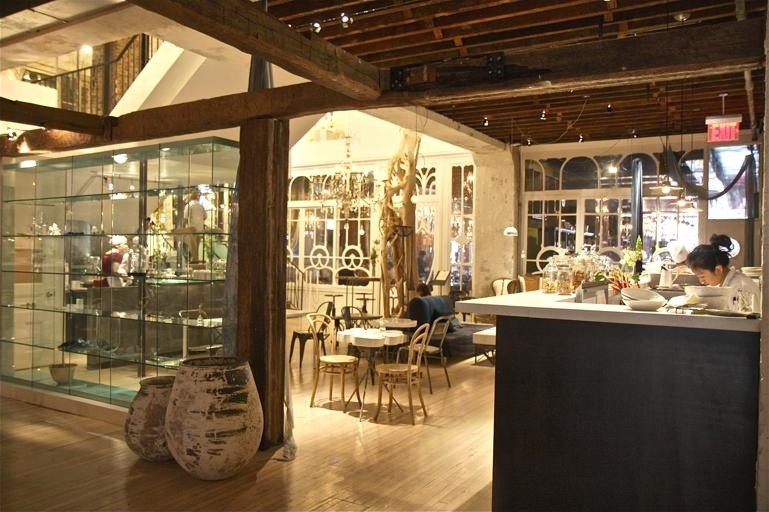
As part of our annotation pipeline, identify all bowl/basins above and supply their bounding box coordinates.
[49,363,78,383]
[699,296,728,309]
[682,285,734,298]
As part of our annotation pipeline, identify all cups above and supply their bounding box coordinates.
[380,318,386,333]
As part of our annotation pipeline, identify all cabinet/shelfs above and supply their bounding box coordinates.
[1,139,244,428]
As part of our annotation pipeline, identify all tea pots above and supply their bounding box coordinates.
[659,270,678,287]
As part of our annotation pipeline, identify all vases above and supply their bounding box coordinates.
[48,362,77,385]
[120,374,179,461]
[161,355,265,484]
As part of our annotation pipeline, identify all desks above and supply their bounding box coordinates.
[471,327,496,364]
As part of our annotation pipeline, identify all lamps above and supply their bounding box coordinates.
[306,135,394,244]
[649,78,684,195]
[681,86,703,214]
[668,80,695,208]
[502,117,521,239]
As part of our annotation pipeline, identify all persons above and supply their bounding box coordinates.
[414,283,432,298]
[684,232,760,307]
[417,250,427,279]
[94,234,150,318]
[172,182,207,266]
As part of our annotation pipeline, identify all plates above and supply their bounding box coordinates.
[619,284,665,311]
[741,265,763,278]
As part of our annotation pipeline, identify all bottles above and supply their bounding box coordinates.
[635,236,643,276]
[544,247,594,295]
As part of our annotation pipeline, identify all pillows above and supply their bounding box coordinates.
[517,273,540,292]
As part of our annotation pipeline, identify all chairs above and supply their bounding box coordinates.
[473,277,520,325]
[289,300,458,429]
[410,295,495,357]
[178,307,223,357]
